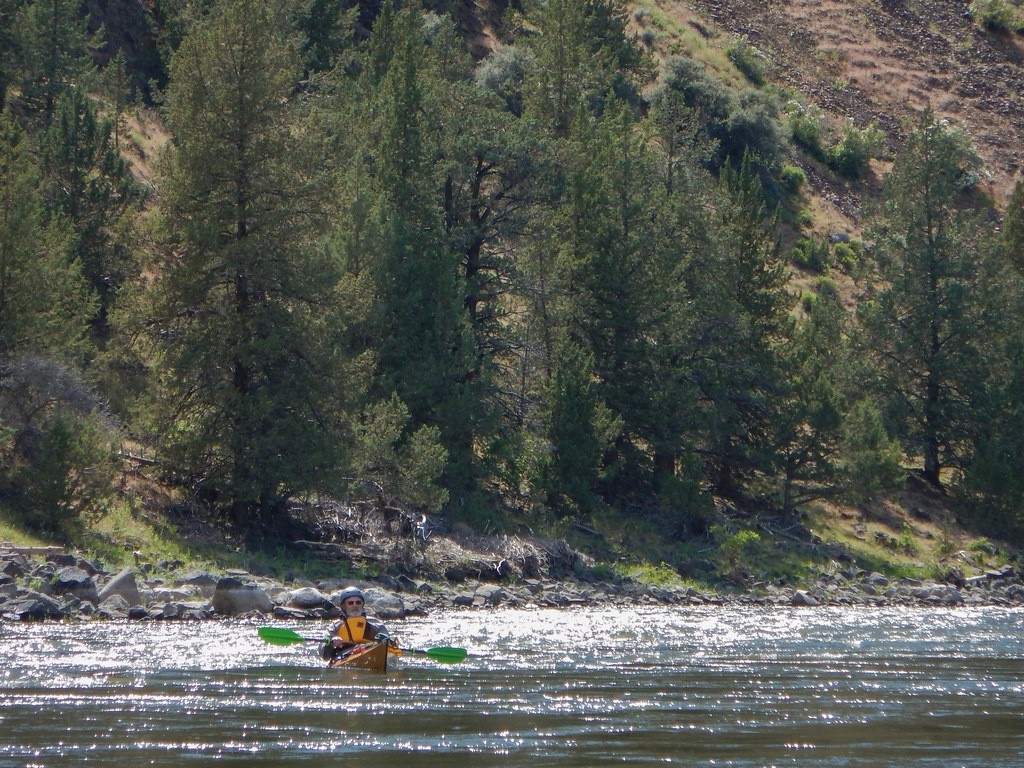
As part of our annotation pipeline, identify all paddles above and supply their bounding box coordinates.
[257,625,467,664]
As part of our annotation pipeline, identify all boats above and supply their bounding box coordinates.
[318,637,389,671]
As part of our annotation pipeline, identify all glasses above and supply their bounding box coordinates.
[343,600,363,605]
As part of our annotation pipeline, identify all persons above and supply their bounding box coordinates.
[317,587,405,666]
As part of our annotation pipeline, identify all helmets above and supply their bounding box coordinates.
[340,587,365,605]
